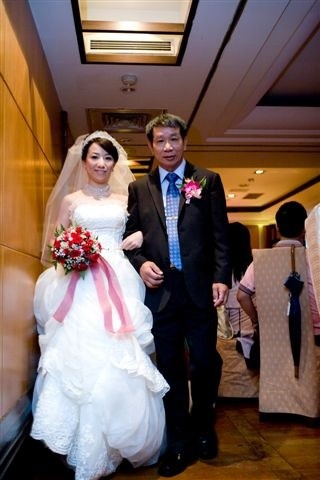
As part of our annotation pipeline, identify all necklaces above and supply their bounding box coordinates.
[83,182,118,198]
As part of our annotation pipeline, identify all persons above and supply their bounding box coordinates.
[123,113,235,477]
[32,130,170,480]
[215,222,254,337]
[237,202,320,345]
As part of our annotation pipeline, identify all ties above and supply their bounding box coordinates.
[165,173,183,271]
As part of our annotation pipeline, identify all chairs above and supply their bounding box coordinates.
[305,204,320,316]
[216,305,259,398]
[251,247,320,417]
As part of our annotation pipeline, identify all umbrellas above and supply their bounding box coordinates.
[284,244,305,380]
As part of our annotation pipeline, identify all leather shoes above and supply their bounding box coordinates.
[196,429,218,460]
[158,444,196,477]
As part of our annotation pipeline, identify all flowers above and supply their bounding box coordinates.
[46,222,101,279]
[175,176,206,204]
